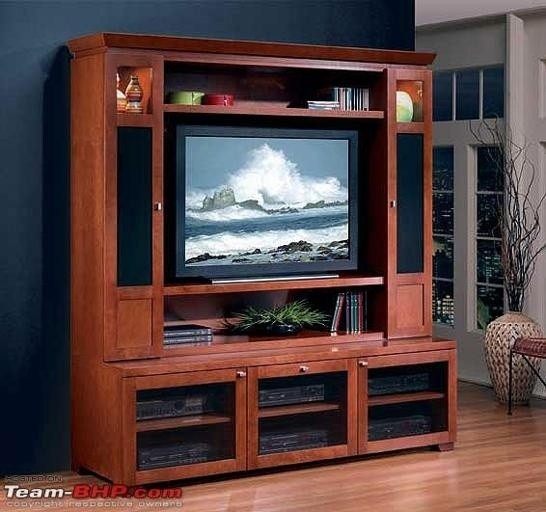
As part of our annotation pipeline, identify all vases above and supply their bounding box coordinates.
[482,312,545,405]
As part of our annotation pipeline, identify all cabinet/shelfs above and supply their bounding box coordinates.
[161,35,385,125]
[363,48,438,343]
[65,32,164,361]
[70,341,457,493]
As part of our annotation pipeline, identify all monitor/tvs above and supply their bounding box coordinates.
[164,126,362,283]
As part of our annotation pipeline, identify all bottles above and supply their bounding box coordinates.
[124,74,143,111]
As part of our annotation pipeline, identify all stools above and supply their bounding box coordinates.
[508,336,546,415]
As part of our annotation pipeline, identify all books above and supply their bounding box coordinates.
[163,324,213,348]
[308,88,369,111]
[330,291,369,337]
